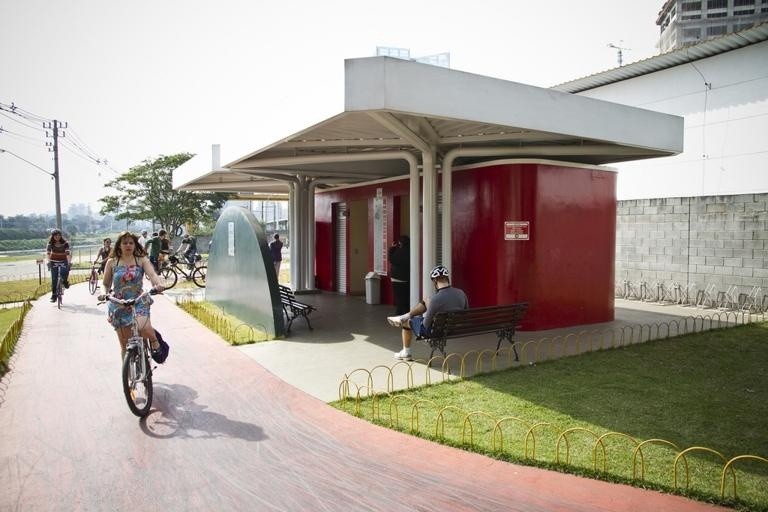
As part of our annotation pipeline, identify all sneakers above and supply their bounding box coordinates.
[150,338,164,356]
[386,316,403,328]
[393,350,412,361]
[63,280,69,288]
[50,294,58,302]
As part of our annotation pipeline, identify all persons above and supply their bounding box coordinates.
[137,229,173,275]
[47,229,71,301]
[94,238,113,294]
[175,233,197,270]
[97,230,164,393]
[268,233,283,280]
[386,265,469,361]
[388,236,410,316]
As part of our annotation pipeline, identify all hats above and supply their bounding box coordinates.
[51,229,62,235]
[141,230,147,235]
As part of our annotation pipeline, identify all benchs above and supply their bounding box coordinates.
[416,302,529,375]
[279,285,317,333]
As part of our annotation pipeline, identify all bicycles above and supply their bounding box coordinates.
[155,256,207,287]
[90,260,98,294]
[47,259,67,309]
[99,286,165,417]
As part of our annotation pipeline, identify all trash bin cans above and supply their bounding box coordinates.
[365,272,381,305]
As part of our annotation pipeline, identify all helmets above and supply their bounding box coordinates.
[159,229,168,236]
[429,266,450,281]
